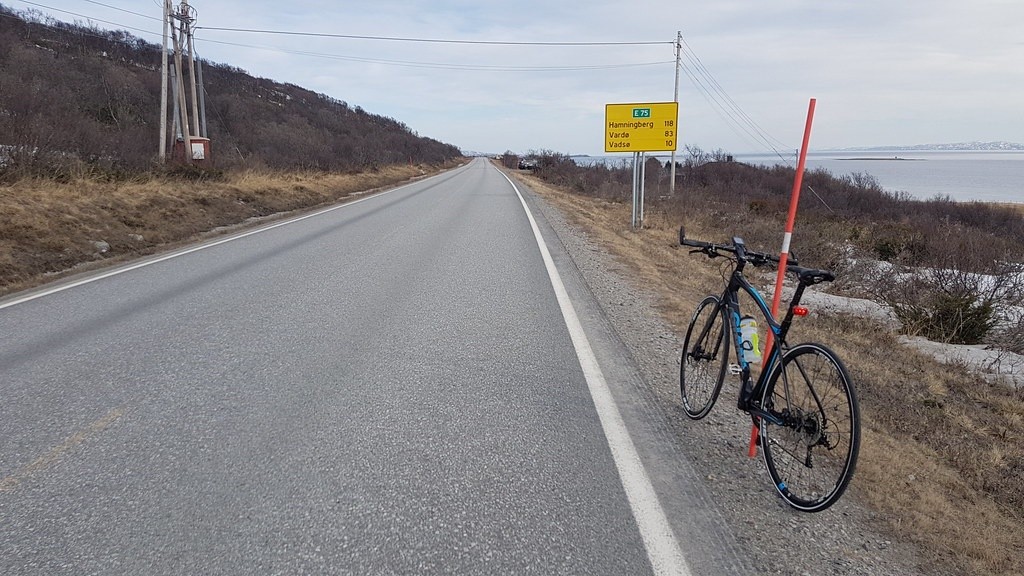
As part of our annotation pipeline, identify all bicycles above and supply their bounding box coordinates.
[677,222,861,513]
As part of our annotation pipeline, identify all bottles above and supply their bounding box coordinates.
[740,314,761,363]
